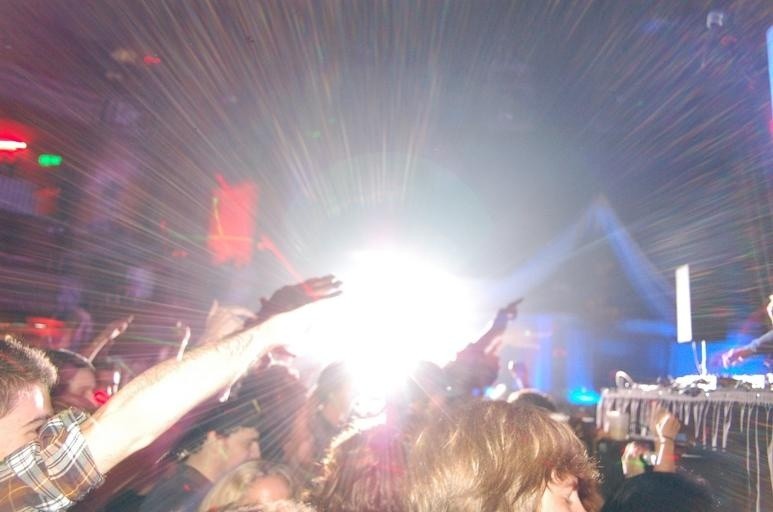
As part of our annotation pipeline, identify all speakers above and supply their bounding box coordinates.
[674,263,727,345]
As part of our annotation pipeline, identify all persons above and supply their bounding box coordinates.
[719,294,772,367]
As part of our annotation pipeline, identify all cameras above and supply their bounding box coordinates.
[640,451,659,467]
